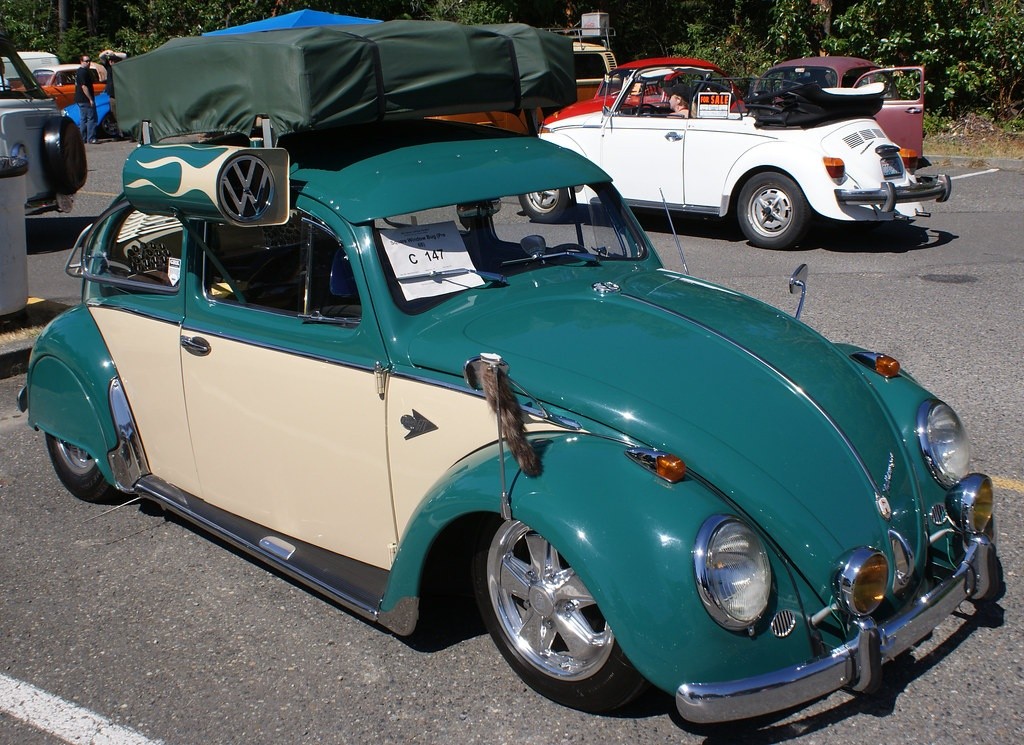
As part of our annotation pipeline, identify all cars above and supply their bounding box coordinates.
[12,63,107,112]
[537,58,745,132]
[424,11,619,130]
[517,55,951,251]
[16,9,1000,724]
[735,55,924,166]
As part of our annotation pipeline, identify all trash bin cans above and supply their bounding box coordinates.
[0,155,29,319]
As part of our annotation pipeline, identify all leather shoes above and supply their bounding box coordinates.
[87,138,101,144]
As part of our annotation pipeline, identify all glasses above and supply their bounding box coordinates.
[83,60,90,63]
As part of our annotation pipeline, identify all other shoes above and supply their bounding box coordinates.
[117,137,124,141]
[130,138,137,142]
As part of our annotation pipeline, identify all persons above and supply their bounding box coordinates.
[661,83,691,118]
[75,55,100,144]
[99,50,127,141]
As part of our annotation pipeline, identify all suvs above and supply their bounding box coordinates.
[0,39,89,221]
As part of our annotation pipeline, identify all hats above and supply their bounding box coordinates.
[664,84,690,103]
